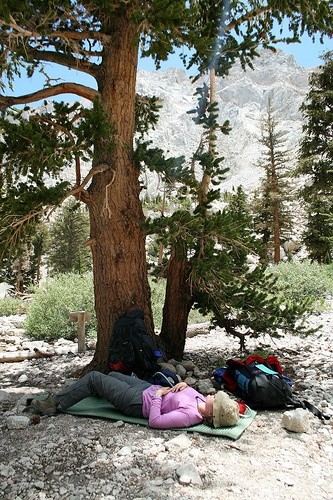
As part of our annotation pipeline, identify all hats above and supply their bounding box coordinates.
[213,390,238,429]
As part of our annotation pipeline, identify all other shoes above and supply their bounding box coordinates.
[26,393,59,417]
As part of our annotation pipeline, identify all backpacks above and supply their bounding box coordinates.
[108,309,178,388]
[212,355,294,409]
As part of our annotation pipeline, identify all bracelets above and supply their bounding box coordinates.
[154,393,161,397]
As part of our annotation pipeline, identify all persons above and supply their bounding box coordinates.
[31,371,241,430]
